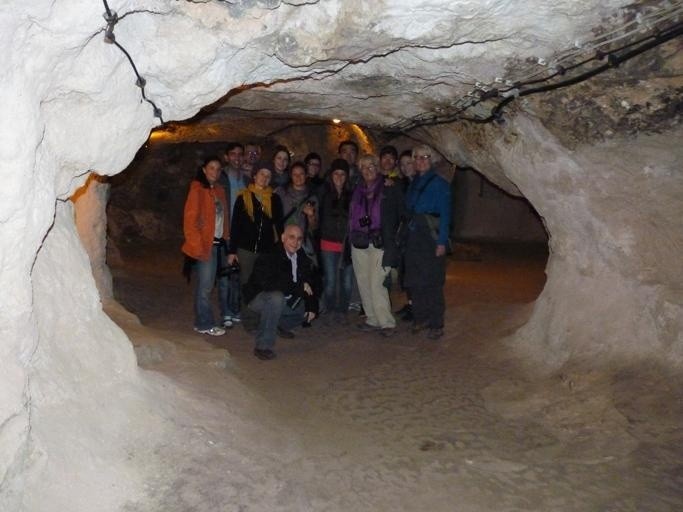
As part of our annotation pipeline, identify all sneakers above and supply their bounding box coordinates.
[194,305,445,361]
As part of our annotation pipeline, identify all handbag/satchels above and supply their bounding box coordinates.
[351,231,383,249]
[236,249,255,284]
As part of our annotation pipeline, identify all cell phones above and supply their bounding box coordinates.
[306,200,312,208]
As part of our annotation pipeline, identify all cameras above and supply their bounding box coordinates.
[285,294,302,311]
[358,216,371,227]
[216,260,241,279]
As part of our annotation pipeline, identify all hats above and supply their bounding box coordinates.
[329,158,349,173]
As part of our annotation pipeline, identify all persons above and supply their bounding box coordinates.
[267,140,414,322]
[181,156,231,335]
[348,155,398,337]
[243,223,321,362]
[227,165,284,285]
[222,142,252,323]
[405,143,451,340]
